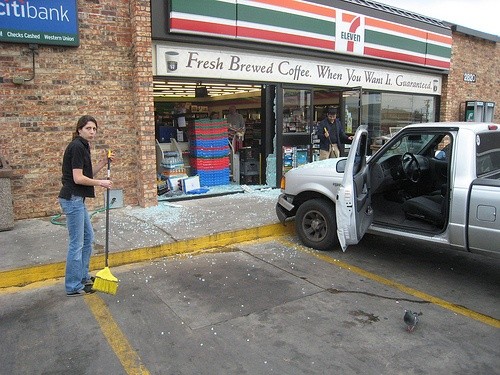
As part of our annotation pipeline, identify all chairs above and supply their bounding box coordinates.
[407,184,448,222]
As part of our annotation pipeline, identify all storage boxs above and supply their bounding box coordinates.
[280,154,294,188]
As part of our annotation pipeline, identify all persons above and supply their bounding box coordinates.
[227,105,245,130]
[58,115,115,297]
[317,108,353,160]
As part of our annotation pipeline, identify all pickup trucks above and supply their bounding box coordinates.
[275,121,500,284]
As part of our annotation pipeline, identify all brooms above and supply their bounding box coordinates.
[323,127,337,158]
[91,149,119,295]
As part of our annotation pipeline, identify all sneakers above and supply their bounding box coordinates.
[67,286,96,296]
[85,277,95,285]
